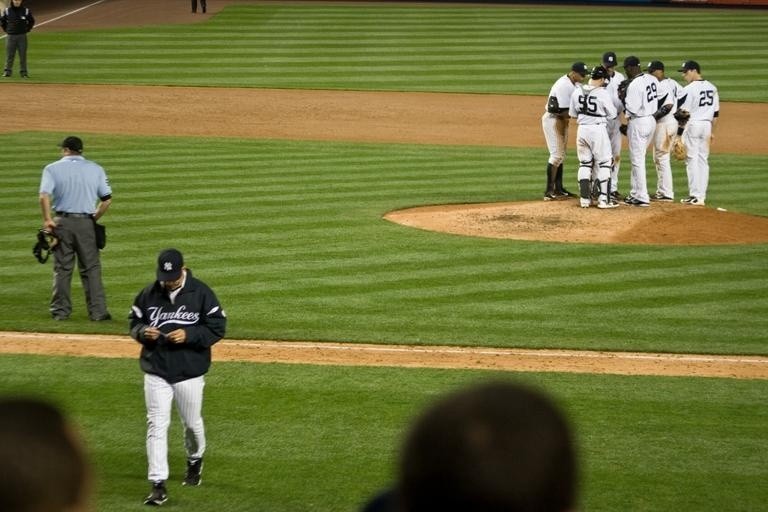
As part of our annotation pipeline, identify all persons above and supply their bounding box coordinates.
[192,0,206,15]
[127,247,228,507]
[540,50,720,209]
[0,0,35,79]
[38,136,115,321]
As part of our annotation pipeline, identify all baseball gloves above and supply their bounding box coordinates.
[672,139,686,159]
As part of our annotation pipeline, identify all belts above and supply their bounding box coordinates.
[57,211,90,217]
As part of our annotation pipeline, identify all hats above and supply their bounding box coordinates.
[157,249,182,281]
[572,51,700,81]
[58,137,83,154]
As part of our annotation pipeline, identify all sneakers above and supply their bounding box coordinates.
[144,483,168,505]
[544,184,705,210]
[186,459,203,486]
